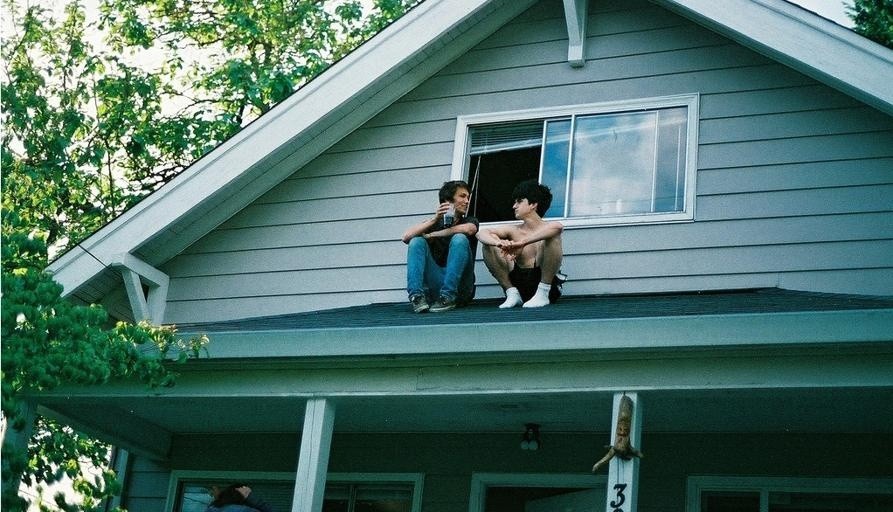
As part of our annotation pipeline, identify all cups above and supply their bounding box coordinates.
[442,203,455,227]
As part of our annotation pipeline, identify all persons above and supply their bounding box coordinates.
[203,483,274,512]
[401,178,568,314]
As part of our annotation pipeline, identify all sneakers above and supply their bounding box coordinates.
[430,294,457,313]
[410,291,429,313]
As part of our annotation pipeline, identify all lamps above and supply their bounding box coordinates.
[519,423,540,450]
